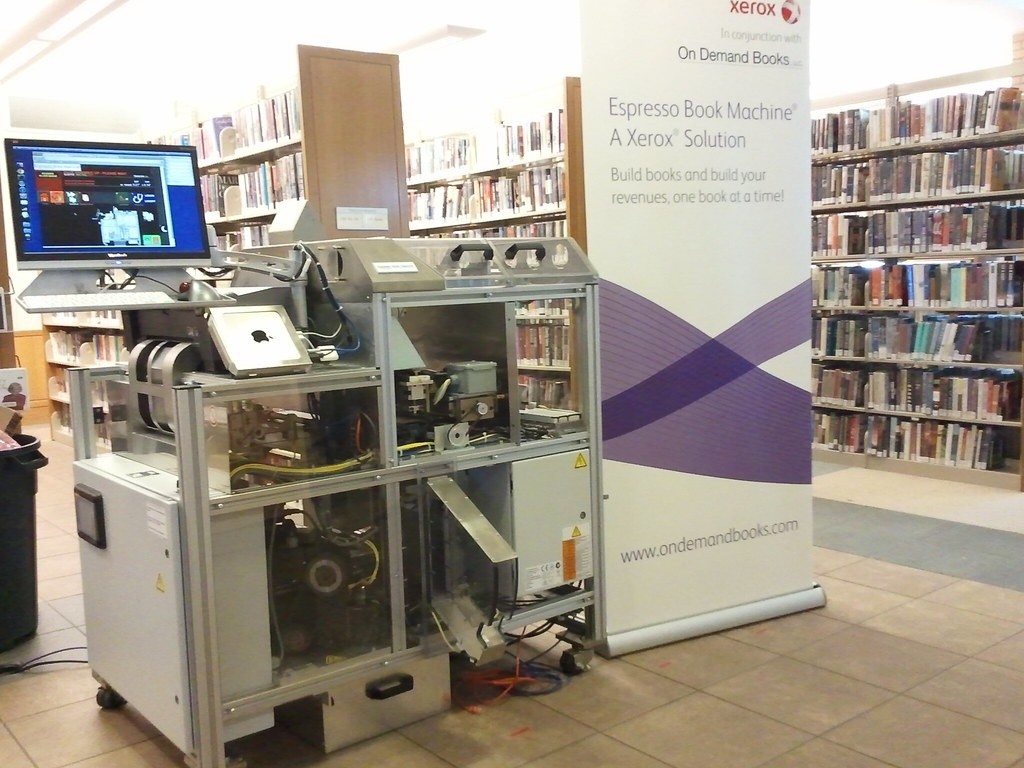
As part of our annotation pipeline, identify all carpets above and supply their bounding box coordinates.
[812,497,1024,593]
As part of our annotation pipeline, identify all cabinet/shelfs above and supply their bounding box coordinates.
[40,44,411,455]
[406,73,586,413]
[809,61,1024,490]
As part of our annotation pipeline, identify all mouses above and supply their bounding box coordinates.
[177,280,221,302]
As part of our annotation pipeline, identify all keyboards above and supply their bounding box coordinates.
[23,291,174,309]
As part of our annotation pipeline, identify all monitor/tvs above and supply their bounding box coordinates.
[3,138,211,271]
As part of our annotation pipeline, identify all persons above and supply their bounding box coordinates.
[3,382,26,410]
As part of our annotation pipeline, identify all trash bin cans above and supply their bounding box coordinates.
[0,434,48,643]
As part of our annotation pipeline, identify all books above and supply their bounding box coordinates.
[403,109,566,256]
[50,309,126,438]
[147,88,305,252]
[516,297,570,408]
[812,87,1024,469]
